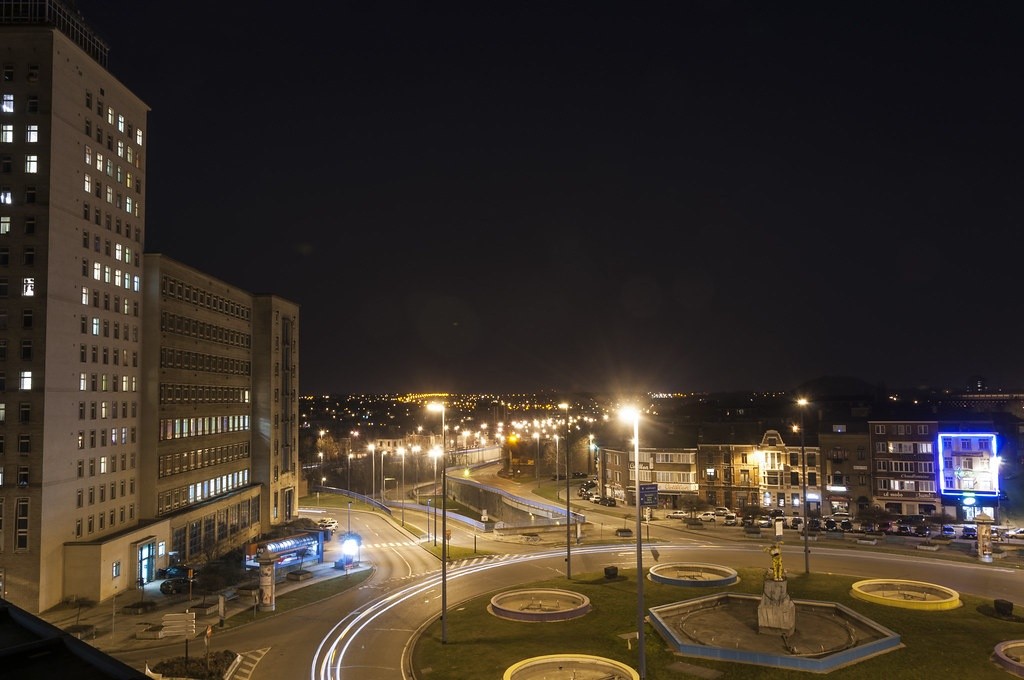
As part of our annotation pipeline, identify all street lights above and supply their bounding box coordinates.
[411,416,584,455]
[188,573,197,600]
[350,430,359,460]
[798,396,812,574]
[535,430,541,487]
[139,583,144,601]
[558,401,574,580]
[554,436,560,482]
[317,451,326,487]
[347,455,354,498]
[397,448,407,530]
[428,402,447,643]
[370,444,376,514]
[318,431,324,442]
[431,450,441,547]
[631,406,649,680]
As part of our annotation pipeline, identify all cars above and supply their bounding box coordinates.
[572,471,586,478]
[159,578,196,595]
[165,567,197,579]
[667,507,1023,540]
[576,476,616,507]
[552,473,565,480]
